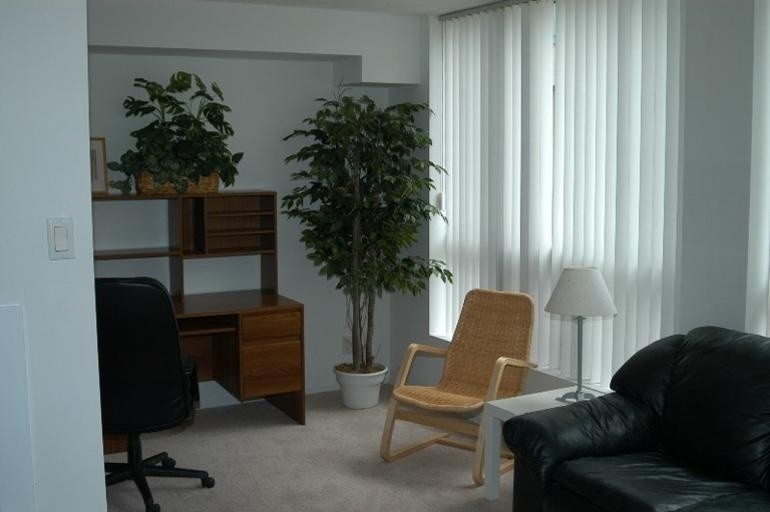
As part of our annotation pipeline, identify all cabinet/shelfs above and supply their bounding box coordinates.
[90,191,306,455]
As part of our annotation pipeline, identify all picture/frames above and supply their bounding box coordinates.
[90,137,109,197]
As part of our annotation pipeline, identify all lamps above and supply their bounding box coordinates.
[545,268,618,402]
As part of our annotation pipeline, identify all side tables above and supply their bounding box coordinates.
[483,386,604,500]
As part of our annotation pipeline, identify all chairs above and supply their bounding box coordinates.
[94,277,215,512]
[381,288,538,486]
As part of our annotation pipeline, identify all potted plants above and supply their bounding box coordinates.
[107,72,244,194]
[279,77,454,411]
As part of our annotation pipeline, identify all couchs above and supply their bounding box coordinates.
[503,326,770,512]
